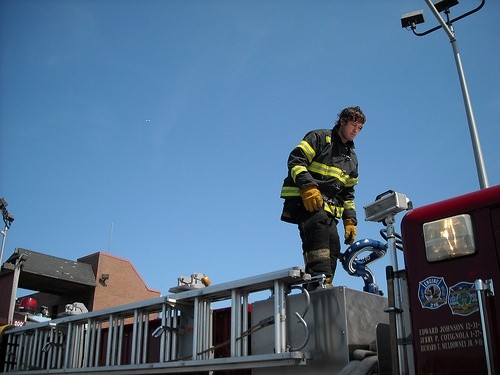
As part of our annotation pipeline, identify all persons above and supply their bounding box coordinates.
[280,106,367,285]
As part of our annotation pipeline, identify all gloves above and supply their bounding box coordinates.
[344,219,356,245]
[301,185,322,212]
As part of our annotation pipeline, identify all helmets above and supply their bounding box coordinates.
[20,297,37,314]
